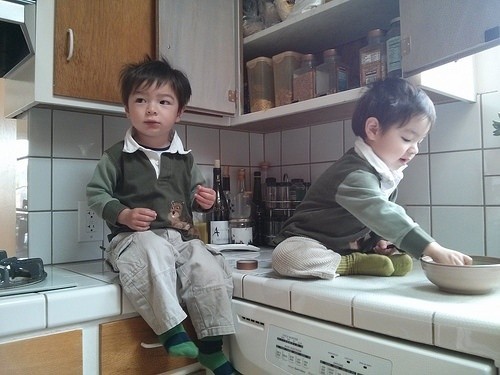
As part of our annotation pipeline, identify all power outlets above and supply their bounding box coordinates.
[77,200,103,242]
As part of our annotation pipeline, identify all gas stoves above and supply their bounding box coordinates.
[0,250,77,296]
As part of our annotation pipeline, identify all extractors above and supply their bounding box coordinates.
[0,0,34,79]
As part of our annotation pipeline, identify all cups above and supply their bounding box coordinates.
[247,51,303,112]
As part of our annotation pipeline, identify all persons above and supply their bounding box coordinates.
[271,75,473,280]
[85,59,240,375]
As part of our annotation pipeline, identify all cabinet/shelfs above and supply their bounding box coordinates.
[55,2,228,127]
[0,306,209,375]
[237,0,500,133]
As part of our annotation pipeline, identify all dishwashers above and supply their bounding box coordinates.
[225,294,499,375]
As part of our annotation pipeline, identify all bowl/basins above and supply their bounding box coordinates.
[419,256,500,295]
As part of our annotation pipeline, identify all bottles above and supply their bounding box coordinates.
[222,164,231,208]
[227,168,253,245]
[250,171,270,246]
[293,47,350,103]
[359,16,403,86]
[207,159,231,246]
[266,178,311,240]
